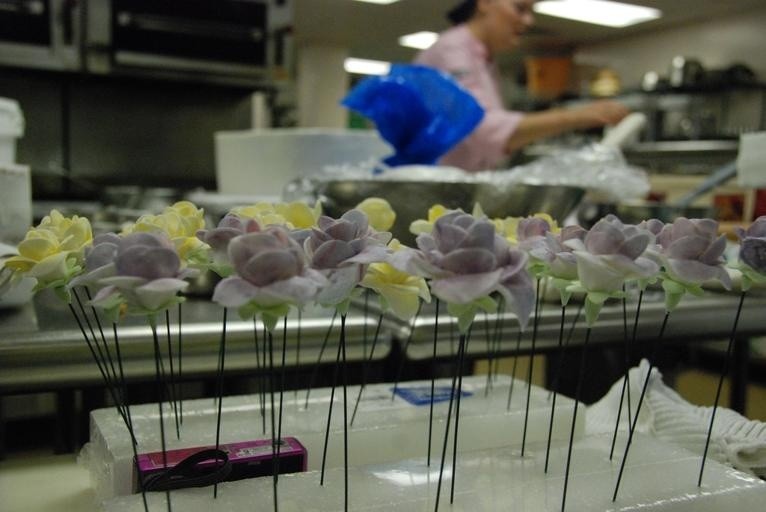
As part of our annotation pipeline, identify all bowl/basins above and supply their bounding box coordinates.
[311,176,584,243]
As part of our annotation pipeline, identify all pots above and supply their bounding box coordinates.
[580,139,741,228]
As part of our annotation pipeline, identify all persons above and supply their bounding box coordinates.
[411,1,631,172]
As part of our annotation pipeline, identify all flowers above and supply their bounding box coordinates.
[0,190,766,511]
